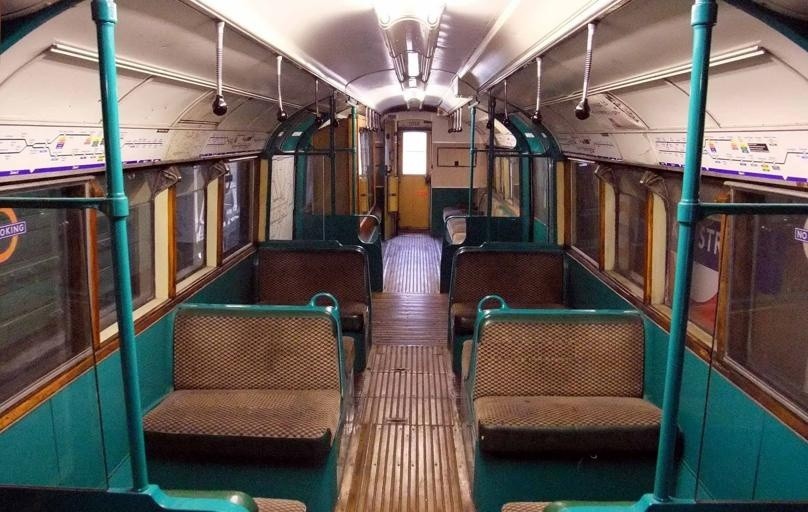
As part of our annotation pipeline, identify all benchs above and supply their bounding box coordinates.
[1,482,307,510]
[466,294,683,510]
[250,241,370,372]
[137,302,348,510]
[440,185,574,376]
[502,501,807,511]
